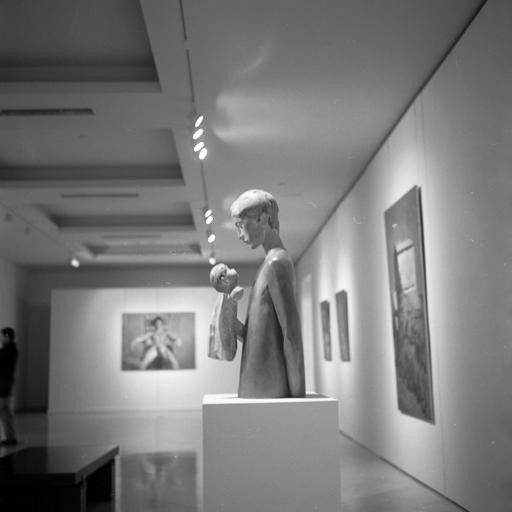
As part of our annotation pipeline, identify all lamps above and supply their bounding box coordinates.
[204,208,216,267]
[191,112,208,161]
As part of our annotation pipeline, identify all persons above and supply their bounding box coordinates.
[229,187,306,398]
[0,326,20,447]
[210,262,244,361]
[133,317,181,369]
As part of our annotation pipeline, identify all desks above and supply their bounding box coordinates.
[0,442,120,511]
